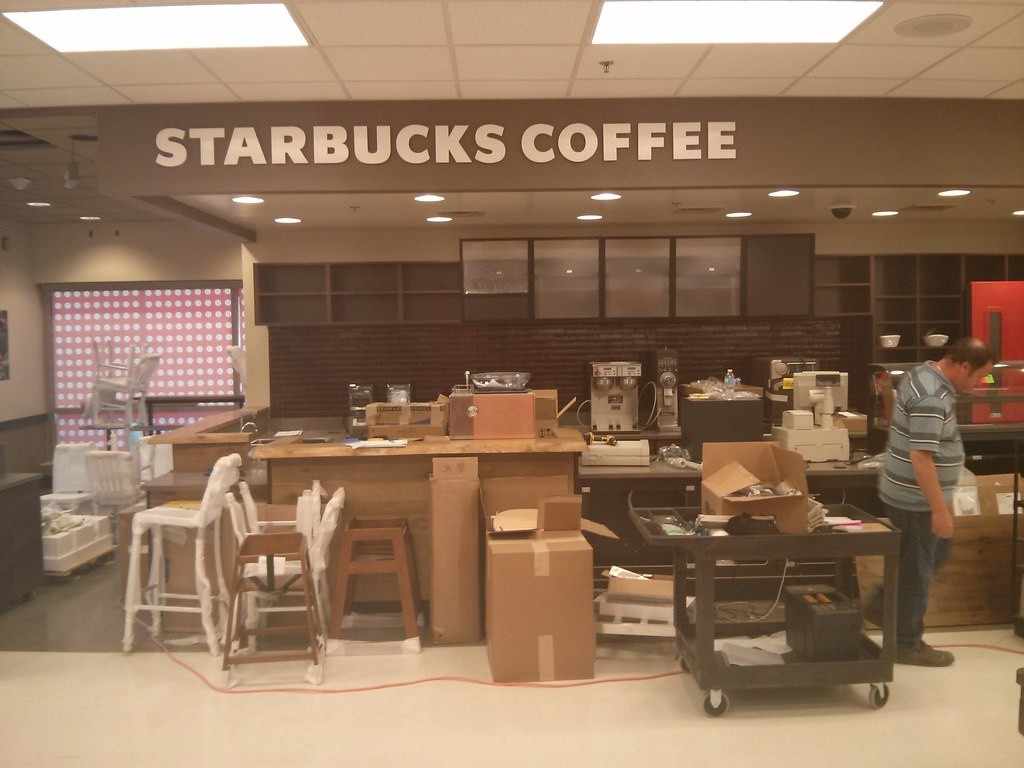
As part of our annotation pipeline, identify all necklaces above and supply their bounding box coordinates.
[935,364,943,373]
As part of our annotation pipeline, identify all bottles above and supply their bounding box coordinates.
[872,395,880,425]
[725,369,735,401]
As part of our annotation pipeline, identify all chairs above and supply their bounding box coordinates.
[122,452,243,656]
[38,442,97,515]
[84,450,140,537]
[80,339,161,433]
[135,434,156,500]
[225,479,346,654]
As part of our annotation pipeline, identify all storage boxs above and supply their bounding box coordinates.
[83,515,112,538]
[42,534,113,572]
[41,531,73,558]
[68,522,96,549]
[364,386,1024,684]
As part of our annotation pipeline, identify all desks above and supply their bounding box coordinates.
[144,410,1024,626]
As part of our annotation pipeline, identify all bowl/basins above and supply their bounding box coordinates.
[881,335,901,347]
[922,334,948,347]
[471,372,530,391]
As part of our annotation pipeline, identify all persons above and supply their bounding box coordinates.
[863,337,998,667]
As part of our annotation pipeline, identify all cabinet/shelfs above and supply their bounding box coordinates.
[531,236,603,324]
[628,503,903,717]
[253,262,462,326]
[814,254,872,318]
[683,477,882,603]
[745,233,815,323]
[602,236,674,323]
[1010,438,1024,639]
[871,253,1024,362]
[674,233,745,323]
[78,393,246,454]
[0,471,45,614]
[461,237,532,324]
[574,439,683,588]
[680,396,764,508]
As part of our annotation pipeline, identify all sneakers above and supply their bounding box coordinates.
[894,640,955,667]
[864,606,885,627]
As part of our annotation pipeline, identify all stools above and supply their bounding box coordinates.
[326,516,428,655]
[219,531,326,689]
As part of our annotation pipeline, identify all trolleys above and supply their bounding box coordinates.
[625,502,902,716]
